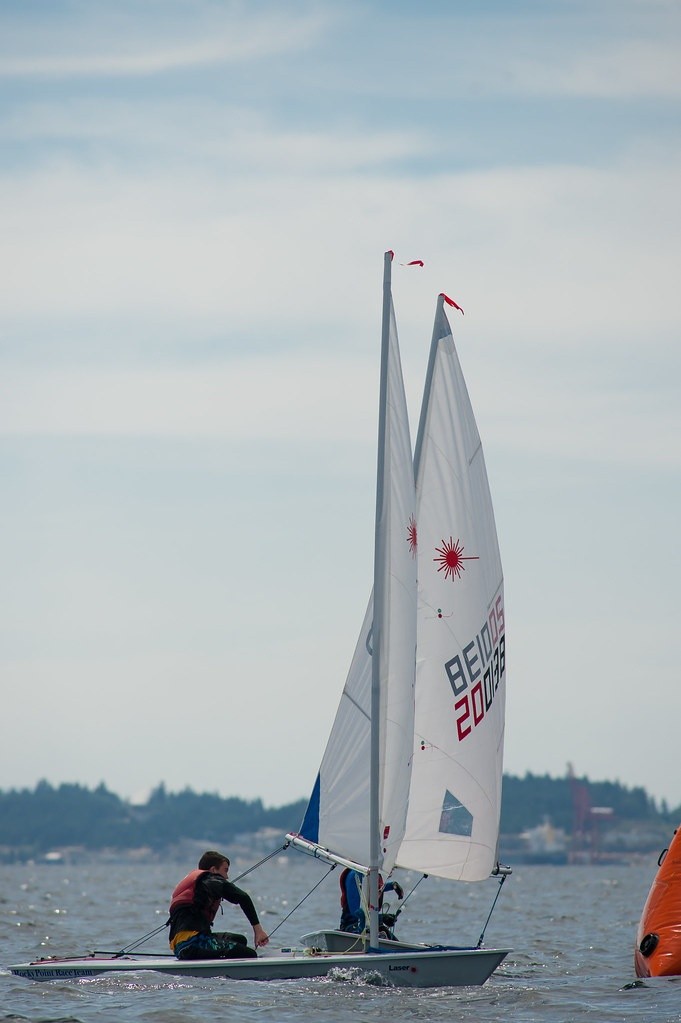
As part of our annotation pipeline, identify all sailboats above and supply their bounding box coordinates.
[8,245,517,987]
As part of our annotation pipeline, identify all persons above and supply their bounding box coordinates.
[168,851,269,961]
[339,861,404,942]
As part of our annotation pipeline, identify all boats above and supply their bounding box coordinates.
[298,929,429,952]
